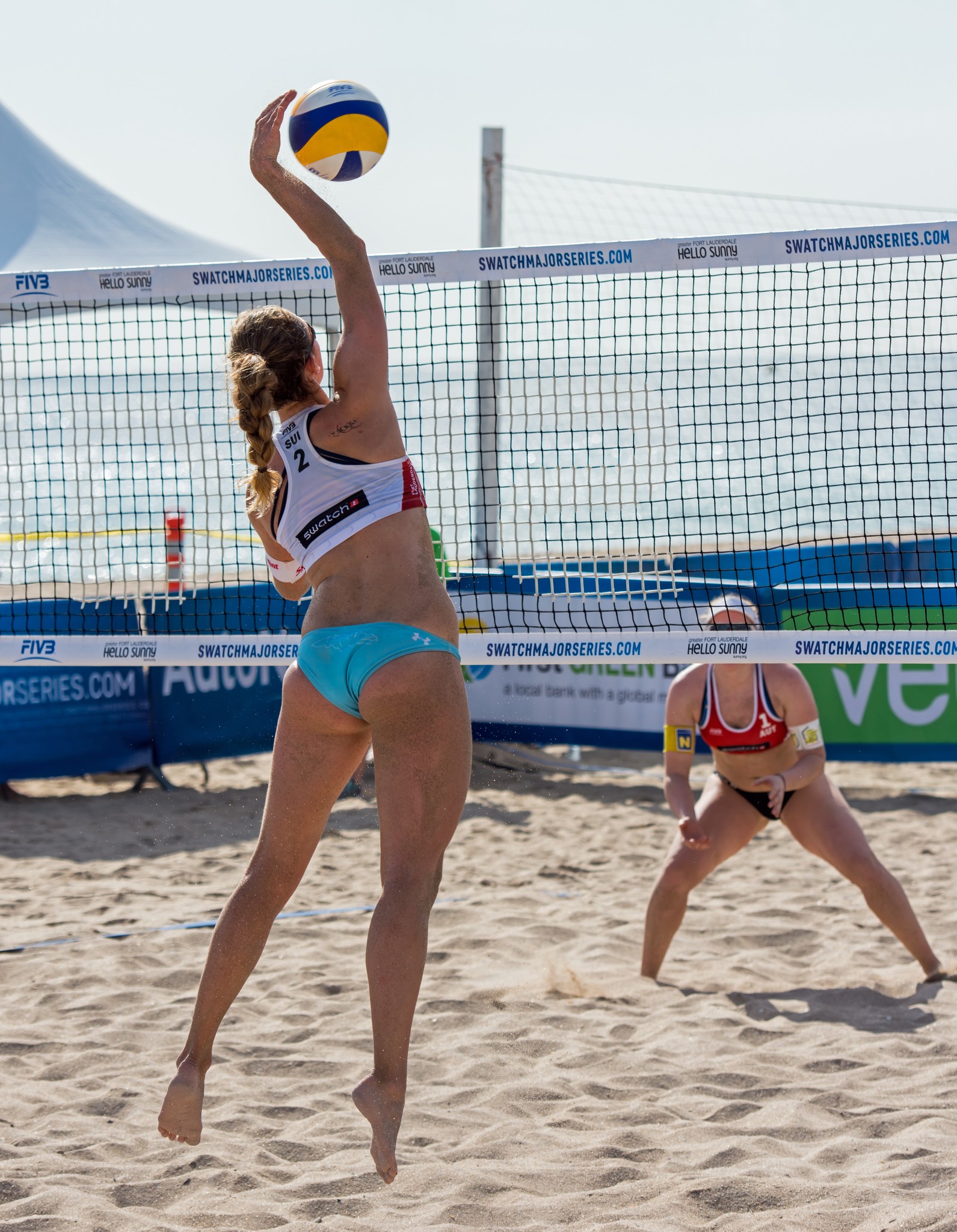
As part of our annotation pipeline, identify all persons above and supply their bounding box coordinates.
[154,90,472,1183]
[640,592,943,980]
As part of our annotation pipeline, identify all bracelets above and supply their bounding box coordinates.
[776,772,789,793]
[788,718,824,752]
[266,554,305,584]
[661,724,696,755]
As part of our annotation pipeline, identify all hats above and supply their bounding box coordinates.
[704,598,761,629]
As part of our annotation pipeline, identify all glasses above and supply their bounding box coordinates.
[710,625,752,633]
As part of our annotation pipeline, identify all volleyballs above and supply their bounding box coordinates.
[288,77,388,181]
